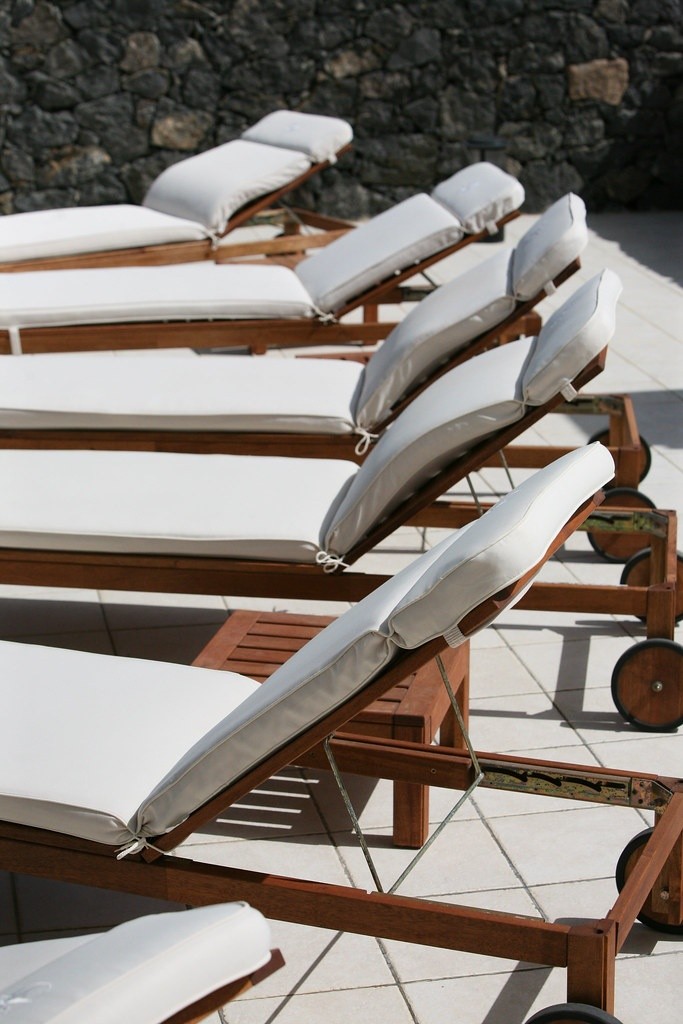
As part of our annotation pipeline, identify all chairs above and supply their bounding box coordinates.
[0,441,683,1023]
[2,195,665,557]
[0,895,287,1024]
[0,158,523,358]
[0,107,350,269]
[0,270,682,732]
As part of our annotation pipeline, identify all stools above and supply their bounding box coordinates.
[183,603,476,845]
